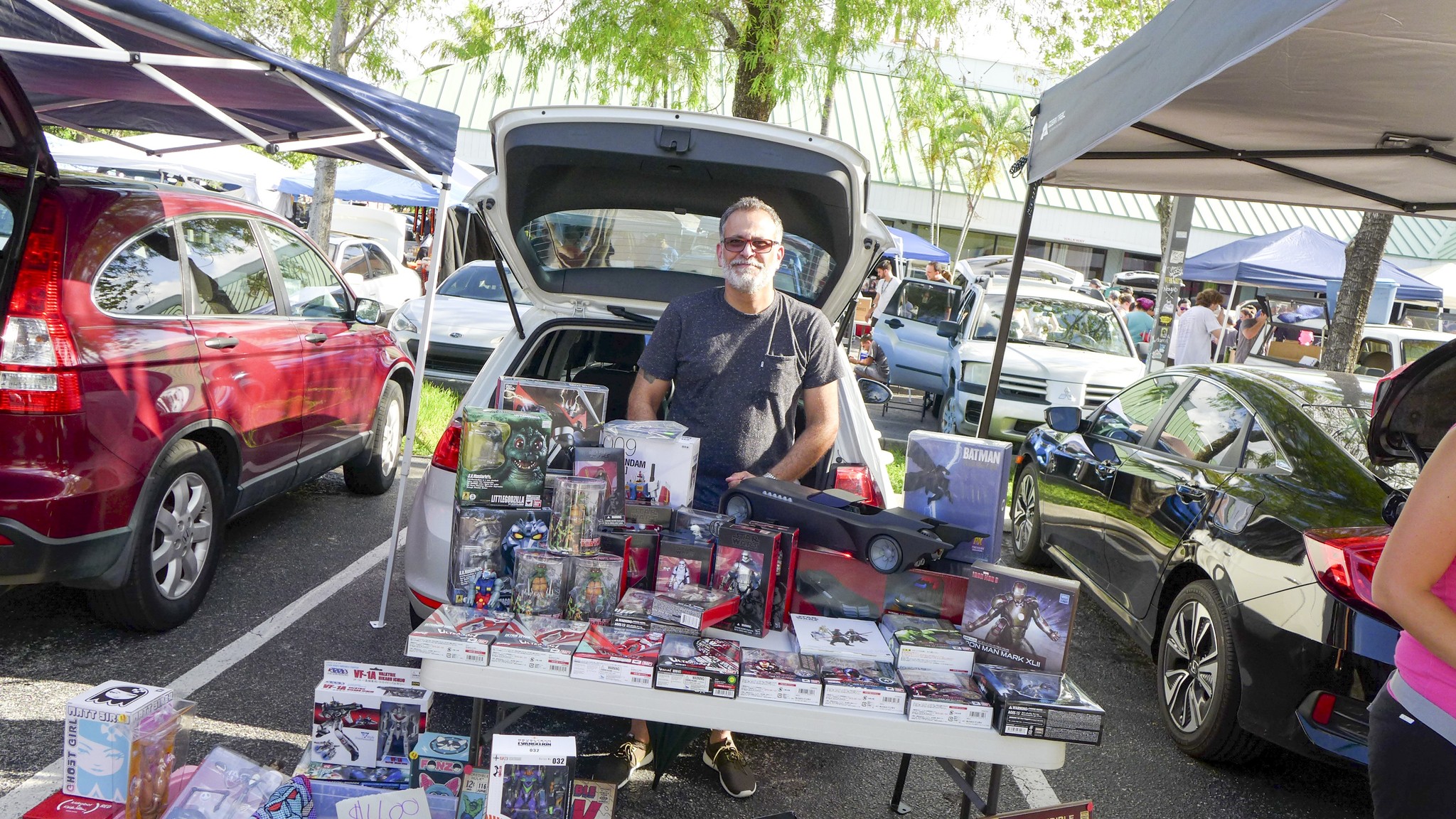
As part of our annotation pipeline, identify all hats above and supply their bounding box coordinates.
[1136,297,1154,310]
[1091,278,1102,287]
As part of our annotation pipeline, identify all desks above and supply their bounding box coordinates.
[422,656,1070,819]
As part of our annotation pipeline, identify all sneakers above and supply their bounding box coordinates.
[592,732,654,790]
[703,733,757,798]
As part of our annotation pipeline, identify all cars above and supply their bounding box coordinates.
[1010,337,1456,776]
[302,228,425,328]
[668,244,807,297]
[387,258,533,395]
[1109,271,1185,305]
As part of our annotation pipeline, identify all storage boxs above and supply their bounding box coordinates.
[19,377,1106,819]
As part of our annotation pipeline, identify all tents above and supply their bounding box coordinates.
[1176,224,1444,363]
[975,0,1456,437]
[875,226,953,284]
[0,2,461,630]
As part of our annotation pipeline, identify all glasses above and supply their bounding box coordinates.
[723,237,779,253]
[1179,305,1189,311]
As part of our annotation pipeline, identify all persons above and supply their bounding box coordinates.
[845,260,953,387]
[1088,278,1269,368]
[1367,421,1456,819]
[587,196,843,798]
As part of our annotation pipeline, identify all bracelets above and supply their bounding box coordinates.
[762,472,779,480]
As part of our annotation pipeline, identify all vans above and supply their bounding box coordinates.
[1244,294,1455,377]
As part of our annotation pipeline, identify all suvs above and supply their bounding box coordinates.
[0,55,419,634]
[873,254,1146,455]
[406,103,895,636]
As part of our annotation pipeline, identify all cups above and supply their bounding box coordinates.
[1214,310,1220,317]
